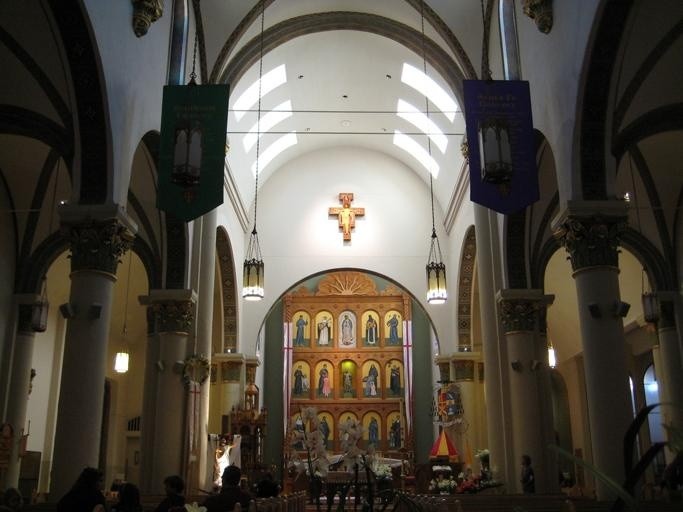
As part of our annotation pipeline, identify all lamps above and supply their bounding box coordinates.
[111,248,135,374]
[31,158,62,333]
[416,3,448,306]
[474,1,515,183]
[168,0,206,184]
[623,151,662,323]
[239,1,268,302]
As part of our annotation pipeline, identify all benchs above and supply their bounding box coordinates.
[391,487,682,512]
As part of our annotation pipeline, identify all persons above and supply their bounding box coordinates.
[361,313,378,344]
[387,313,400,344]
[150,475,189,512]
[105,480,143,511]
[211,436,242,489]
[55,466,107,511]
[199,464,251,511]
[315,313,331,346]
[166,495,188,512]
[0,487,24,512]
[519,453,536,494]
[290,412,402,456]
[293,313,308,346]
[340,313,354,344]
[339,201,353,236]
[290,360,402,401]
